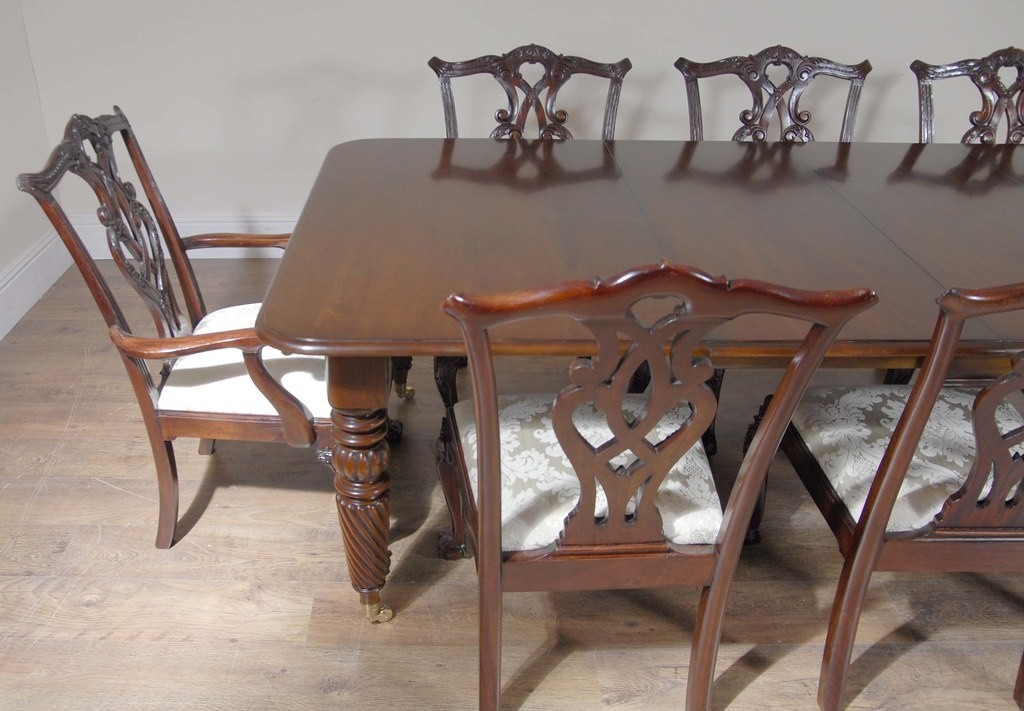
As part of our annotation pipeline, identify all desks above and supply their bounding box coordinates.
[257,132,1024,625]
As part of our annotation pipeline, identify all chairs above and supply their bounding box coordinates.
[674,44,877,450]
[741,278,1024,711]
[430,139,623,194]
[664,141,852,198]
[886,47,1024,385]
[887,143,1024,199]
[14,105,412,550]
[430,44,635,402]
[435,258,880,711]
